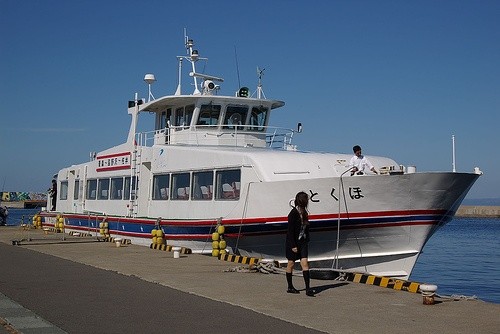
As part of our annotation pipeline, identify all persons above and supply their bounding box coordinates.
[349,145,379,176]
[286,192,318,297]
[50,179,57,211]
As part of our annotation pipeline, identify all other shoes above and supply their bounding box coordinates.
[53,208,56,210]
[50,208,54,211]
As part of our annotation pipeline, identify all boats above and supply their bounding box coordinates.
[41,36,483,283]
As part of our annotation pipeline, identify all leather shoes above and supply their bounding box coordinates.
[287,288,300,294]
[306,289,314,296]
